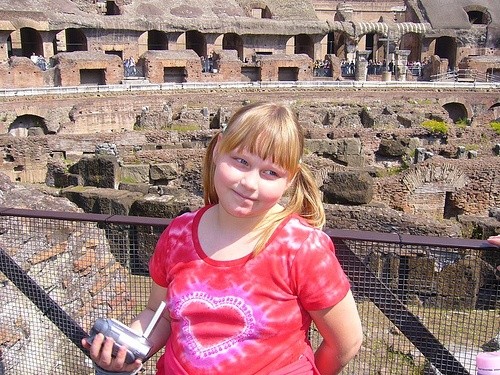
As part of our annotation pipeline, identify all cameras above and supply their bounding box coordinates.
[86,300,166,364]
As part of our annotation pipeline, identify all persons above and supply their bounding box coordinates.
[124,56,136,77]
[31,53,44,64]
[201,55,213,72]
[239,53,260,64]
[82,100,364,375]
[312,56,429,77]
[487,234,500,271]
